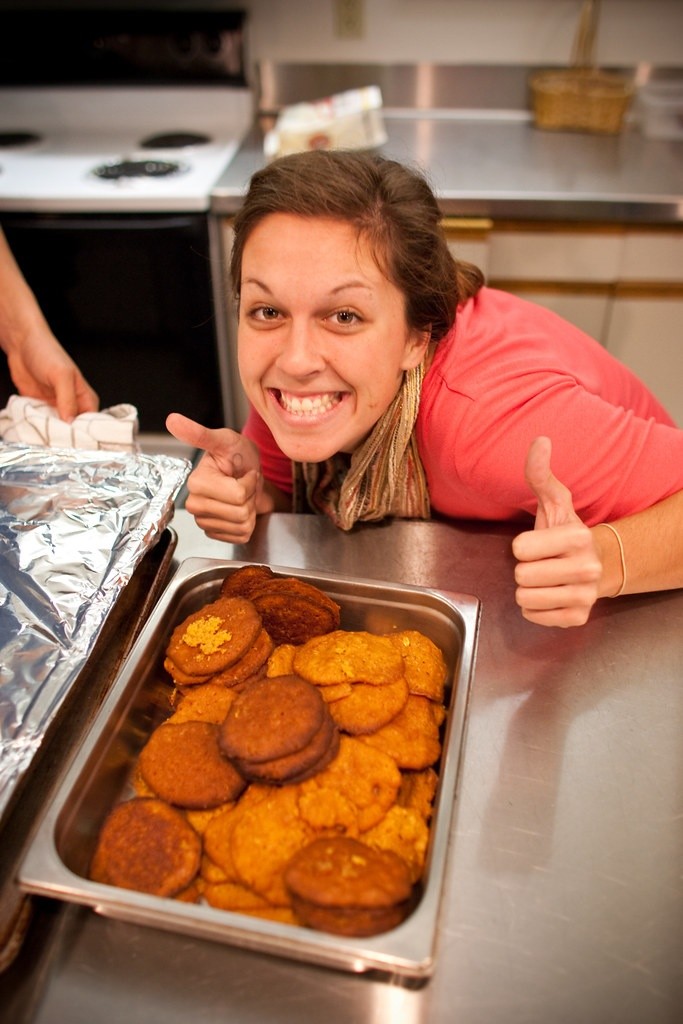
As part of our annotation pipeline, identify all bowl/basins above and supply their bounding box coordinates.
[639,85,683,140]
[18,559,483,982]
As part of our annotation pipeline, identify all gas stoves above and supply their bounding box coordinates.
[0,85,256,216]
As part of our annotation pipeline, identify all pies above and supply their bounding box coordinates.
[85,567,446,935]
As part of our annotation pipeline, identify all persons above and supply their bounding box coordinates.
[0,227,99,424]
[166,150,683,628]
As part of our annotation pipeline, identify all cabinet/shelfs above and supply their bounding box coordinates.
[219,211,683,436]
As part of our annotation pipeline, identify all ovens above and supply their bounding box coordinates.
[0,211,224,435]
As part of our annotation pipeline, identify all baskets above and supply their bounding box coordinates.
[527,42,632,137]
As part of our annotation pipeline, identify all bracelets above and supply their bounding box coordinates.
[596,523,626,598]
